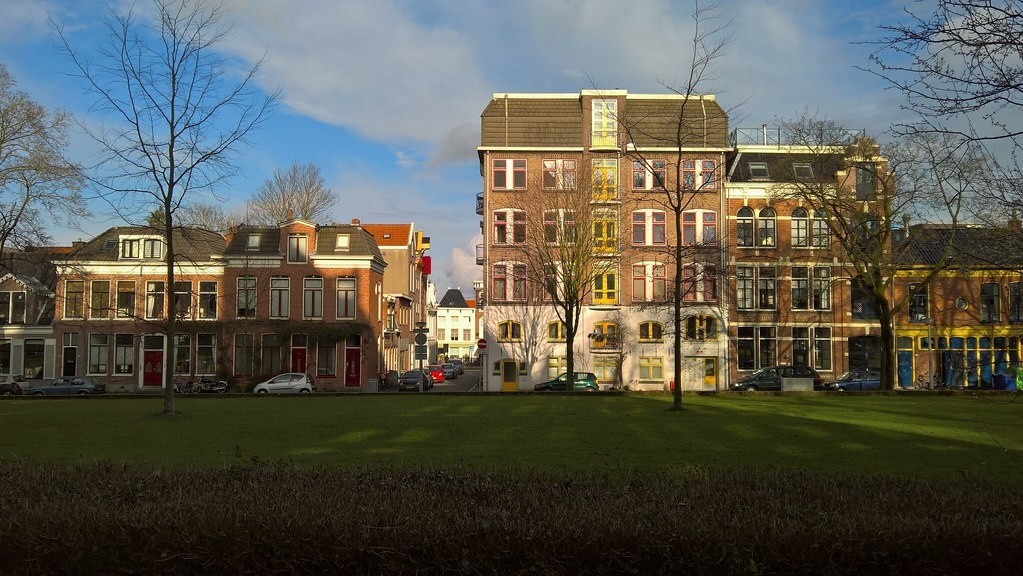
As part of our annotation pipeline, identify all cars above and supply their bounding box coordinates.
[253,373,317,394]
[730,365,824,392]
[412,368,433,389]
[0,374,29,396]
[424,365,445,383]
[534,372,600,392]
[823,371,881,391]
[448,360,464,375]
[442,363,457,379]
[398,370,428,391]
[29,376,106,395]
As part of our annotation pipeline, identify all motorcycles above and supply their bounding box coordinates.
[191,376,228,393]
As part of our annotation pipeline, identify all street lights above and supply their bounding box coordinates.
[415,322,426,392]
[925,318,934,390]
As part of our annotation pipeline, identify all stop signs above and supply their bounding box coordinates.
[477,339,487,349]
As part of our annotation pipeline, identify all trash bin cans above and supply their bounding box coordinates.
[992,373,1016,390]
[670,380,675,391]
[1006,366,1023,390]
[387,370,398,387]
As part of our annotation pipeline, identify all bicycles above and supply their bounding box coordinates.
[914,372,942,390]
